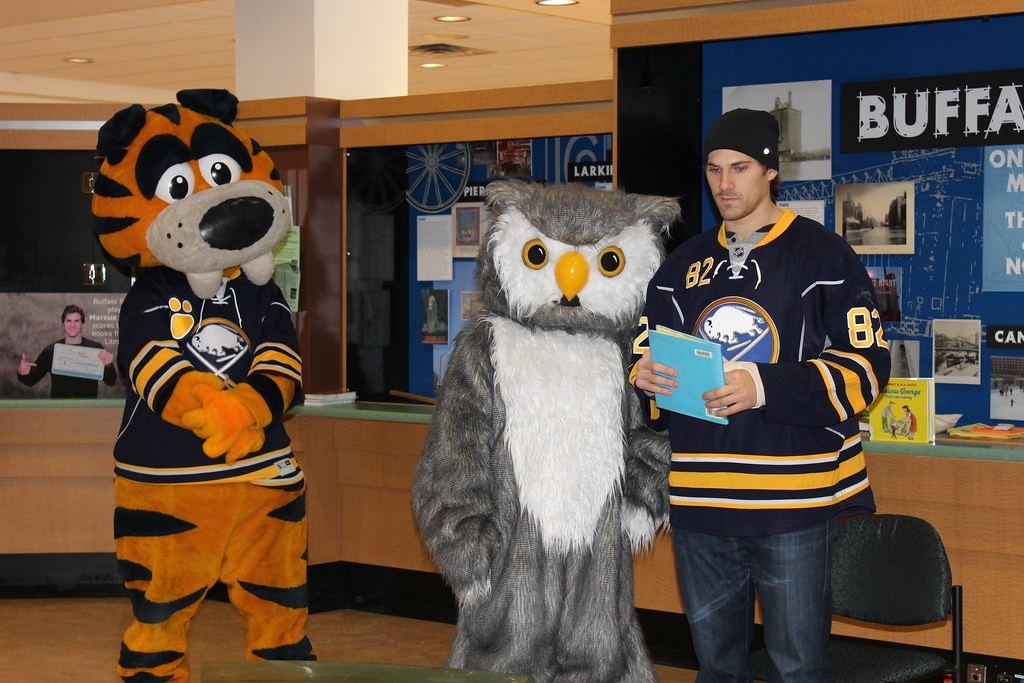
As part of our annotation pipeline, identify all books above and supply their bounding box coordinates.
[302,392,356,405]
[647,324,730,426]
[866,377,937,446]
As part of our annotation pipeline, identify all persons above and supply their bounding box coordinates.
[15,303,118,401]
[622,104,893,683]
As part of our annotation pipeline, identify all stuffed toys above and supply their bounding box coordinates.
[411,178,678,682]
[85,87,320,683]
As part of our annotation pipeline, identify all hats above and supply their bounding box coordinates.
[704,108,780,171]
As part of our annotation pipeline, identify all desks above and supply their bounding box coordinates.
[0,396,1024,664]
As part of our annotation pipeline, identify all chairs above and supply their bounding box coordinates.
[824,514,987,683]
